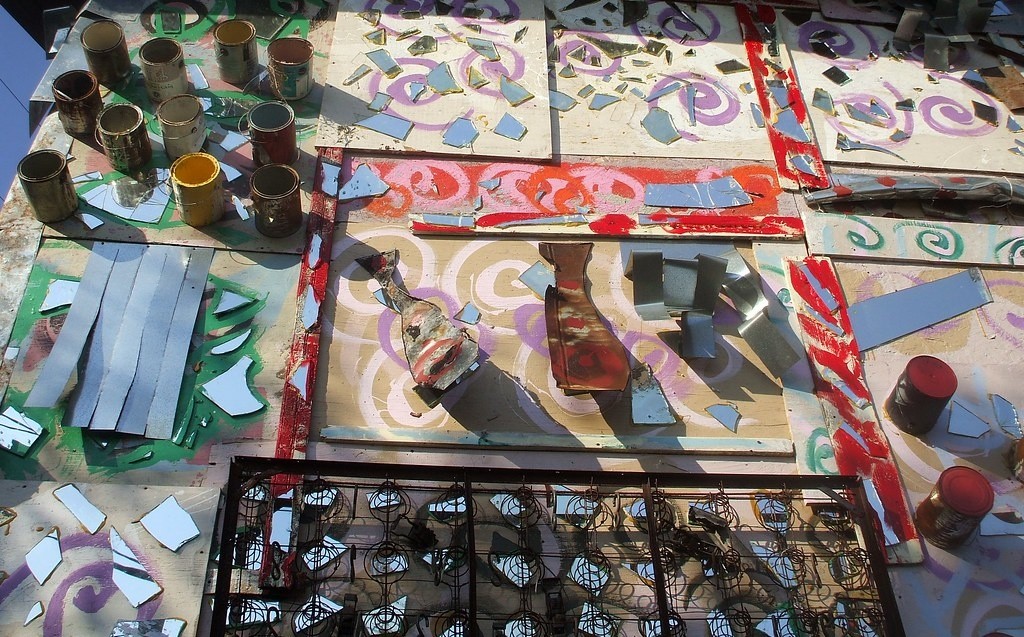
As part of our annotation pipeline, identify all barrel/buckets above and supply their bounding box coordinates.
[249,163,302,238]
[139,37,188,103]
[267,37,314,100]
[170,152,224,227]
[247,100,297,167]
[52,70,104,134]
[79,20,131,83]
[156,94,206,157]
[17,149,79,223]
[94,103,151,173]
[213,19,258,83]
